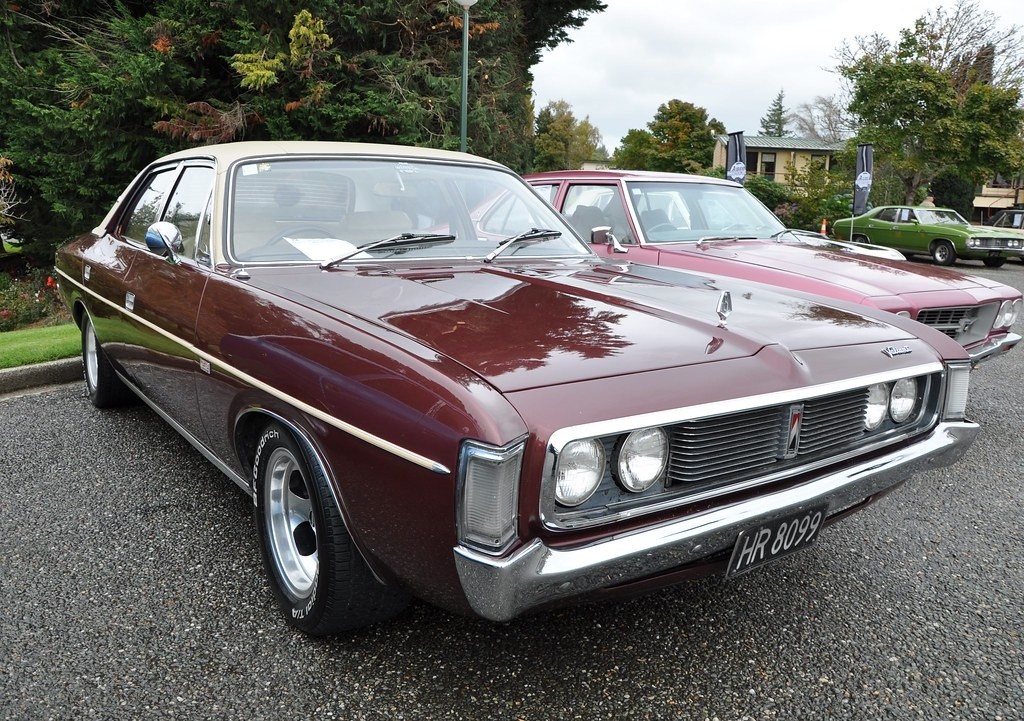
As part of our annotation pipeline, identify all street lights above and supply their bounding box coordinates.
[453,0,481,152]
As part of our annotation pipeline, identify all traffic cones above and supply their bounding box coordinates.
[819,217,828,236]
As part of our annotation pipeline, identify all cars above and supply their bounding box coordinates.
[422,169,1024,368]
[831,206,1023,266]
[54,140,981,636]
